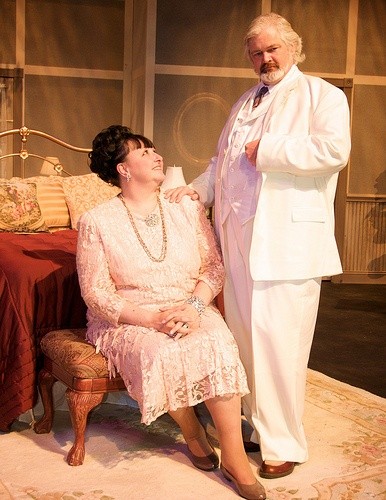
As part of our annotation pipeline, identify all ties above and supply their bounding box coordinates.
[252,86,269,108]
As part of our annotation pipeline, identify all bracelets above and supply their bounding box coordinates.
[185,296,205,315]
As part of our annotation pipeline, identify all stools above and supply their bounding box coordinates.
[33,328,127,466]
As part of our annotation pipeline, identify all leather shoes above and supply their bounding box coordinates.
[189,436,219,472]
[259,460,295,479]
[243,440,261,452]
[221,461,267,500]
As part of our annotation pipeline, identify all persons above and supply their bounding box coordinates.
[76,126,266,500]
[164,13,351,479]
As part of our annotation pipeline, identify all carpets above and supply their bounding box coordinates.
[0,367,386,500]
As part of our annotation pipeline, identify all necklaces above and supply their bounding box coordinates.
[116,189,167,262]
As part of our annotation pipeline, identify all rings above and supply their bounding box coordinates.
[183,325,188,328]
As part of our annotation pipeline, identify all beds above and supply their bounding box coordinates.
[0,125,117,435]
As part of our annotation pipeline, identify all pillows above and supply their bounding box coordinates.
[0,173,121,233]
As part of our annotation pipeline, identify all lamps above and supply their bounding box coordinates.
[160,164,187,193]
[41,157,62,176]
